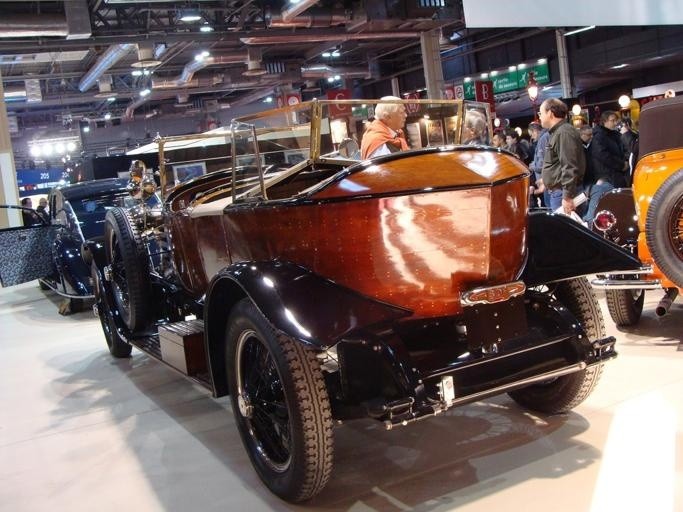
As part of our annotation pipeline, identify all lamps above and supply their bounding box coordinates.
[27,135,81,157]
[571,104,584,128]
[492,116,502,127]
[618,94,631,119]
[526,71,541,107]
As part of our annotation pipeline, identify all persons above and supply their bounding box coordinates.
[453,95,637,224]
[360,95,408,161]
[21,198,42,228]
[37,197,50,222]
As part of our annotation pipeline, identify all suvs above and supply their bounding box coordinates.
[77,94,655,505]
[586,88,683,332]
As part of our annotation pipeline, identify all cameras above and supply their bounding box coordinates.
[617,120,629,130]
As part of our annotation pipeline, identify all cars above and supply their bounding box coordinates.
[1,175,163,313]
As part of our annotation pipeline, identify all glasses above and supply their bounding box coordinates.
[537,111,546,116]
